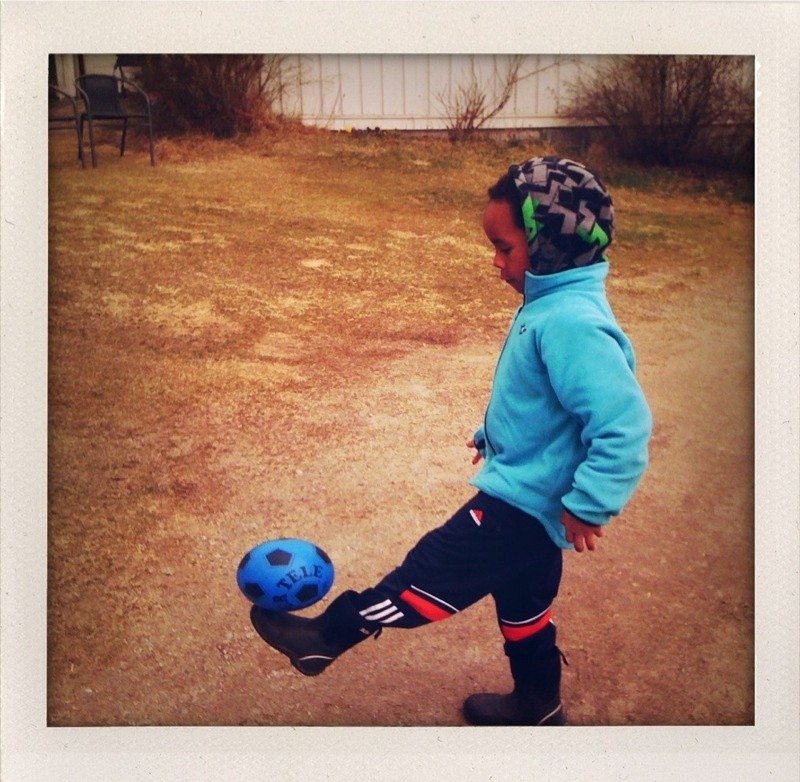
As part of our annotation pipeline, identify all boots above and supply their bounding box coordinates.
[463,642,569,726]
[248,590,383,677]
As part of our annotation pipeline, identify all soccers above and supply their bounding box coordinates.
[236,537,335,613]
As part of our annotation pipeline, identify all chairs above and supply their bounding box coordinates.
[74,74,155,166]
[48,85,87,168]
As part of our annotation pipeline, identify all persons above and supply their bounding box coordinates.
[250,156,654,726]
